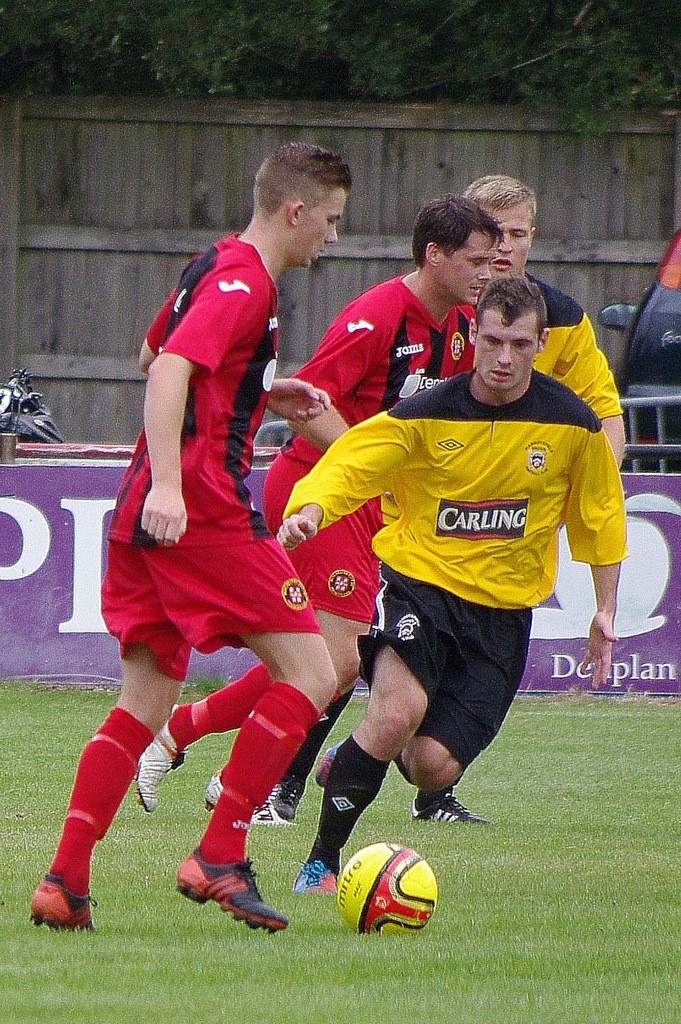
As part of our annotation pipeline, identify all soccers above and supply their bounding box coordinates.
[336,842,437,933]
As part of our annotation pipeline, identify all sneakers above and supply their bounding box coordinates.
[204,769,224,811]
[252,775,306,828]
[292,858,338,897]
[316,738,348,787]
[176,848,288,934]
[133,703,189,812]
[410,792,493,825]
[30,874,97,933]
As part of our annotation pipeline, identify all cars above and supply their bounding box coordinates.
[596,228,681,476]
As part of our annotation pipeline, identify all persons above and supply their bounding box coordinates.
[134,195,507,824]
[254,175,627,824]
[276,273,628,895]
[28,141,351,932]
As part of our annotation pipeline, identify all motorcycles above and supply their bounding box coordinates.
[0,366,64,445]
